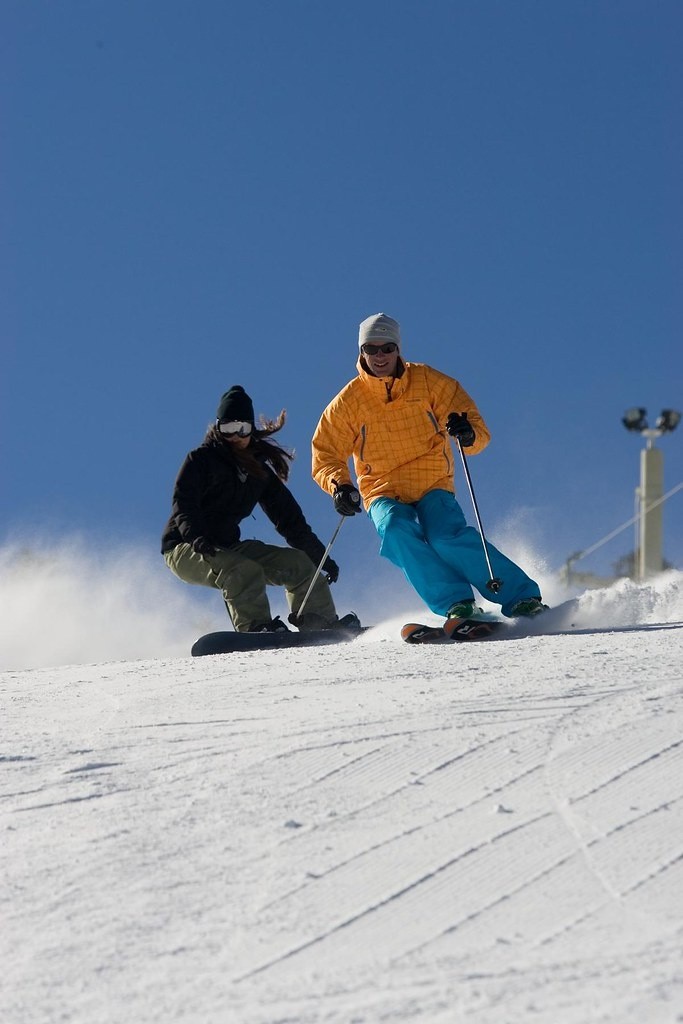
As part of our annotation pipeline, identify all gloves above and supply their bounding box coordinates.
[331,478,361,516]
[446,412,475,443]
[188,532,216,557]
[322,559,339,585]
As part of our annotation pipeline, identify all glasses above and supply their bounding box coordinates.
[361,343,397,355]
[215,419,254,438]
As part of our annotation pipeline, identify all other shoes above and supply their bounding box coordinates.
[448,600,475,619]
[298,613,322,632]
[250,615,289,632]
[511,597,546,617]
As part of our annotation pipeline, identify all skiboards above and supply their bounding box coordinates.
[399,598,579,643]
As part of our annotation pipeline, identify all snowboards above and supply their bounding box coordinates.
[189,626,376,656]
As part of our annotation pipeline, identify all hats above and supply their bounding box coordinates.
[217,385,254,426]
[358,313,401,356]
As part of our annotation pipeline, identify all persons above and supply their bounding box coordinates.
[161,385,360,630]
[311,311,542,617]
